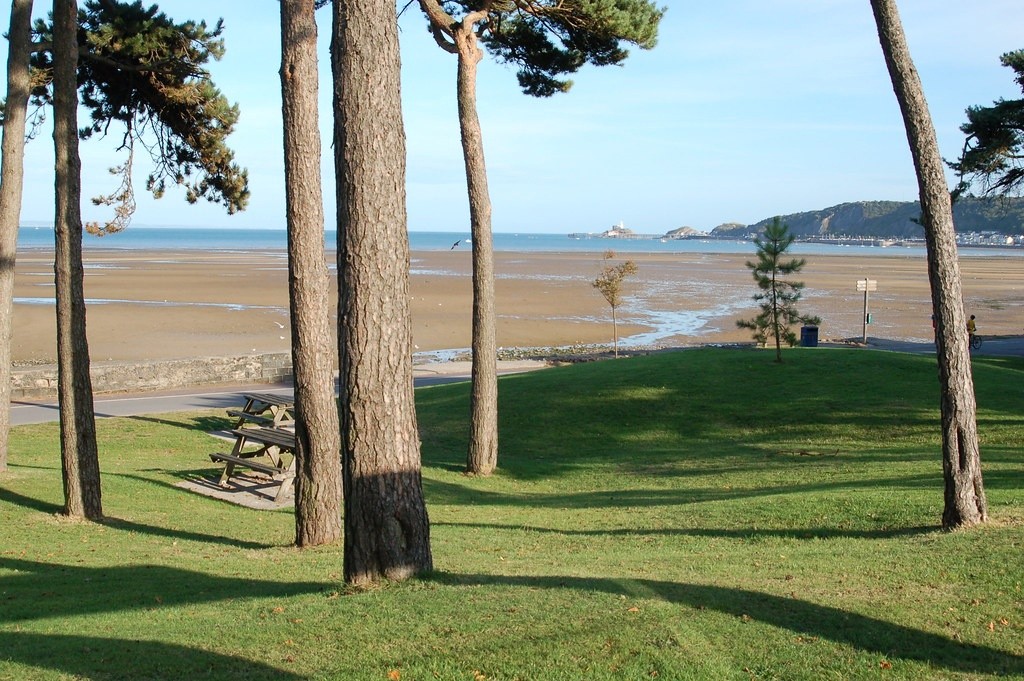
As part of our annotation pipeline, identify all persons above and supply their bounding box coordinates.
[966,315,976,349]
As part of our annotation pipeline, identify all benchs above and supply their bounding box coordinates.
[226,410,275,427]
[209,453,295,482]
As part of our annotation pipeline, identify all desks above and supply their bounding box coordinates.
[218,427,295,502]
[234,393,294,429]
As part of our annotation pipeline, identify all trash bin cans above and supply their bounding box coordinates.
[801,326,818,347]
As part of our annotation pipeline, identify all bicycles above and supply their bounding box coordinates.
[968,329,982,349]
